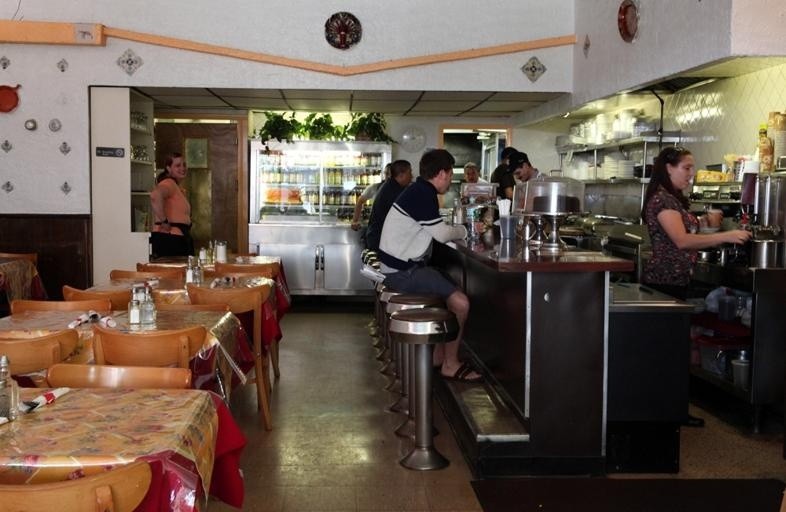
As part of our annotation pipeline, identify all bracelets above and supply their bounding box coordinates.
[162,219,169,224]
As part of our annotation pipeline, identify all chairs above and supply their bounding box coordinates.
[0,253,285,512]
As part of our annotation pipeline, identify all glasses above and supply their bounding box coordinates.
[668,146,685,164]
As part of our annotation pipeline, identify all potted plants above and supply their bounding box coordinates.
[251,112,400,152]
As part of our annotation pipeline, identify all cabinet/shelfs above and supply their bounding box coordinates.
[555,136,680,224]
[89,86,154,287]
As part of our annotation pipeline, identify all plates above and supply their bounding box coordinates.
[602,153,653,179]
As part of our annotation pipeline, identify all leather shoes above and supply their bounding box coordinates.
[681,415,705,427]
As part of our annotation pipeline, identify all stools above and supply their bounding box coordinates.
[361,247,459,471]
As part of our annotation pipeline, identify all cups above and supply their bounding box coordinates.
[131,110,151,162]
[708,209,723,229]
[500,215,516,239]
[766,111,786,168]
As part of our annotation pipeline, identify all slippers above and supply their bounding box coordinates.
[432,359,485,382]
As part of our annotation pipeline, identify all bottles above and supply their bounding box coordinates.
[186,240,227,286]
[759,136,773,172]
[128,282,157,327]
[258,149,384,220]
[0,354,20,426]
[450,197,481,246]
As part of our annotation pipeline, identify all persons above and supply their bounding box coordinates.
[640,147,754,428]
[506,152,546,182]
[365,160,413,250]
[463,162,488,193]
[490,148,519,220]
[150,153,195,258]
[378,150,487,383]
[351,163,392,231]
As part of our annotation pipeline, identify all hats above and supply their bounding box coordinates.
[506,151,527,175]
